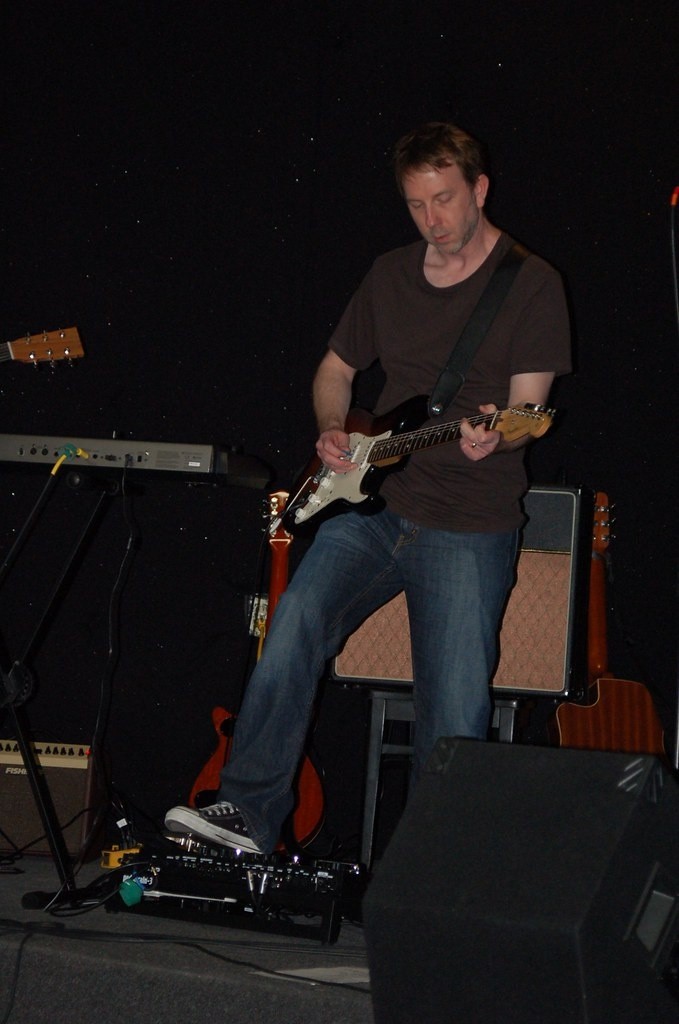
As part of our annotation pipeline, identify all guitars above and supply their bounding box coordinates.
[545,487,671,770]
[288,396,557,530]
[182,488,331,859]
[0,320,89,369]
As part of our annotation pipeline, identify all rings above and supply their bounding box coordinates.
[472,444,476,448]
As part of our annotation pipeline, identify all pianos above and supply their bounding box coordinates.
[0,429,237,478]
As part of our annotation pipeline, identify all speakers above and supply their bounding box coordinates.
[328,484,597,698]
[0,739,108,861]
[362,736,679,1024]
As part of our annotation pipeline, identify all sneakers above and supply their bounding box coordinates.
[164,801,265,854]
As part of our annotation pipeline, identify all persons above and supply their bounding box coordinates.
[162,119,573,853]
